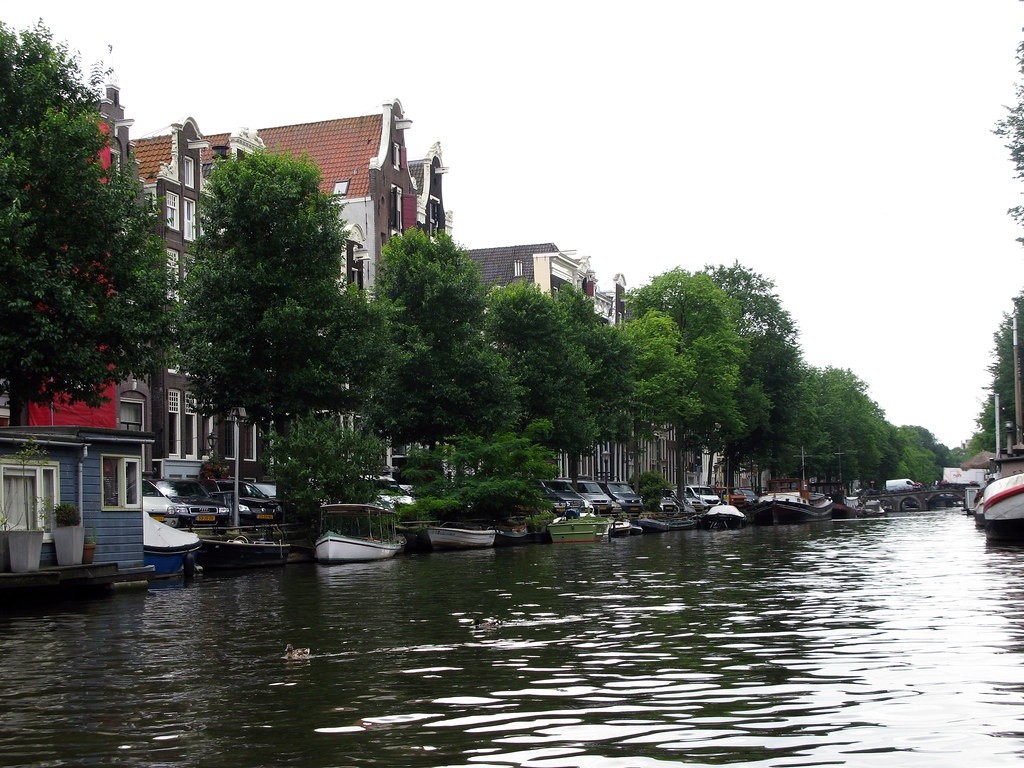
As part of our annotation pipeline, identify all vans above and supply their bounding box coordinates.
[886,478,915,492]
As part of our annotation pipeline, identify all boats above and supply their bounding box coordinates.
[392,533,408,554]
[974,470,1024,546]
[610,518,696,537]
[832,498,885,517]
[699,504,746,530]
[192,538,291,572]
[748,445,833,524]
[495,527,530,542]
[143,511,200,578]
[426,524,496,547]
[313,503,402,563]
[547,515,616,543]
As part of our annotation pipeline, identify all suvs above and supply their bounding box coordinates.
[143,478,229,535]
[558,478,643,514]
[201,478,284,528]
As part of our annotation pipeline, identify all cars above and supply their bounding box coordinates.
[245,482,289,508]
[657,488,686,517]
[527,484,567,518]
[536,479,590,514]
[357,475,416,510]
[713,486,759,509]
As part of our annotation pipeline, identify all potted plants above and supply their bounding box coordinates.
[51,501,85,565]
[0,436,49,572]
[83,527,97,564]
